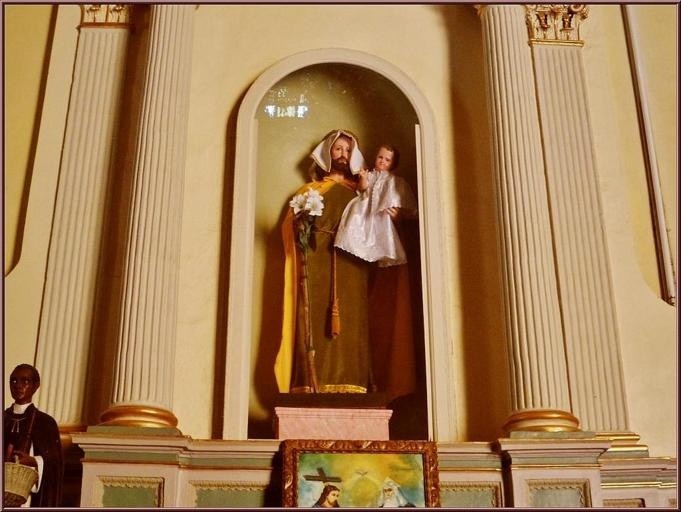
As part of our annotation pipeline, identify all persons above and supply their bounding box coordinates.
[377,475,415,507]
[2,363,64,507]
[312,485,340,508]
[333,142,417,268]
[274,130,417,401]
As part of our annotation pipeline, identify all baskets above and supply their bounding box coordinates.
[3,455,39,507]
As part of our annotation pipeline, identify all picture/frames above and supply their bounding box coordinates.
[281,440,440,509]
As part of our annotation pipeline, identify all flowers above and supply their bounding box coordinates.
[290,189,324,348]
